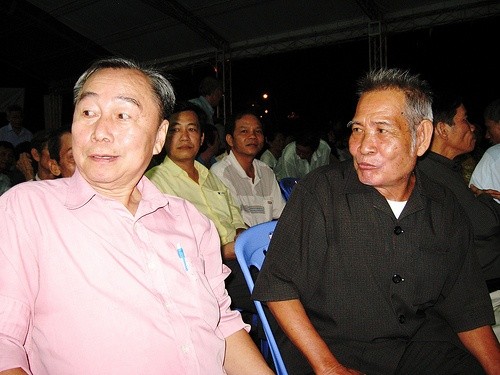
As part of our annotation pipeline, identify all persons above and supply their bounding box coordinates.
[0,59,276,375]
[0,78,500,375]
[253,68,500,375]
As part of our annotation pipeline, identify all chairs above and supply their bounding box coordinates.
[279,177,299,201]
[235,220,290,374]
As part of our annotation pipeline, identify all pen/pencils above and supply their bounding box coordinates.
[174,241,190,273]
